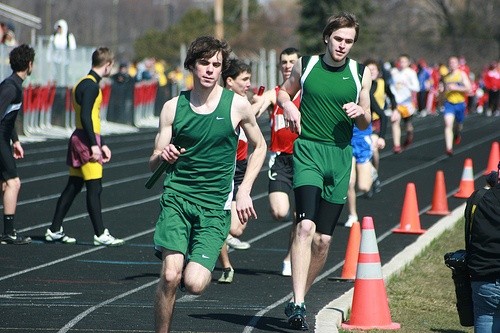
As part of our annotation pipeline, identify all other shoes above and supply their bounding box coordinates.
[366,190,373,198]
[373,167,378,182]
[477,107,483,113]
[495,111,500,117]
[487,109,492,116]
[446,150,453,155]
[1,231,32,245]
[218,267,234,283]
[282,261,292,276]
[44,226,77,245]
[392,146,401,153]
[406,135,413,145]
[225,234,250,249]
[371,134,386,149]
[421,111,427,117]
[344,214,358,227]
[284,300,309,330]
[455,136,462,144]
[374,180,381,193]
[93,229,125,246]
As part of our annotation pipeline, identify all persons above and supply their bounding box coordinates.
[149,36,268,333]
[109,56,194,91]
[218,47,304,283]
[465,161,500,333]
[0,44,35,246]
[0,20,17,47]
[46,18,76,98]
[345,54,500,228]
[277,10,372,330]
[45,47,127,248]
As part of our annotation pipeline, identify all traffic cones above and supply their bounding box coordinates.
[391,182,430,234]
[483,141,500,177]
[327,221,362,282]
[340,216,401,330]
[426,171,453,215]
[453,157,476,199]
